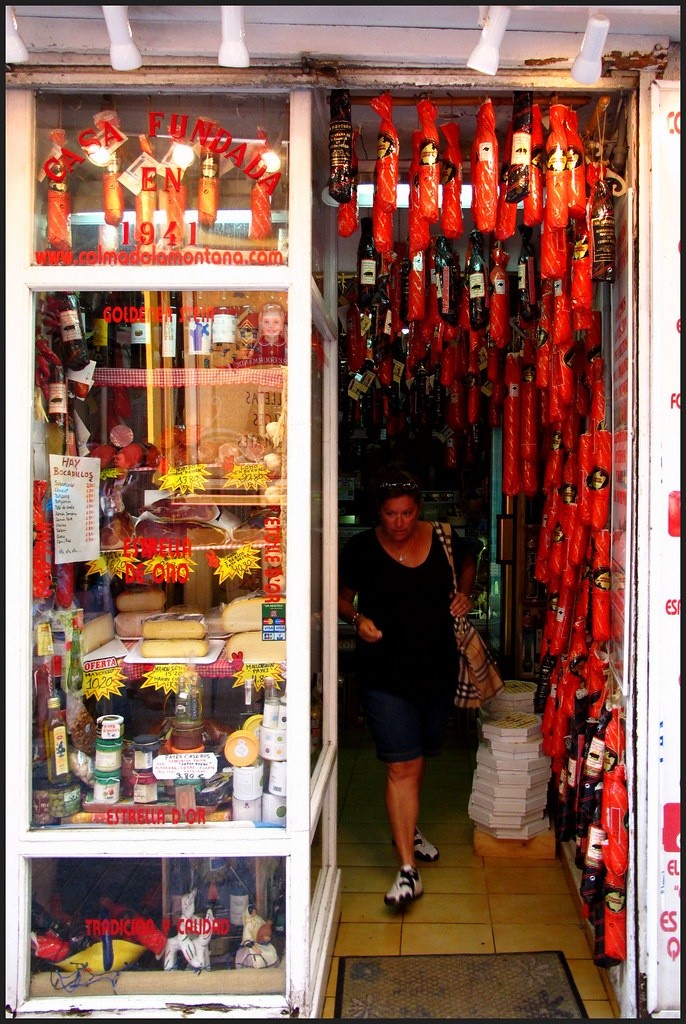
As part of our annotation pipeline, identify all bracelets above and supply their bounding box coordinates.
[352,613,363,630]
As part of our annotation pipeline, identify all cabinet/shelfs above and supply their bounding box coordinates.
[1,73,344,1021]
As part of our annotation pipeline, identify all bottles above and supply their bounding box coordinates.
[261,677,279,726]
[43,696,70,784]
[229,858,251,928]
[174,661,202,724]
[49,331,69,425]
[58,290,89,372]
[92,290,237,370]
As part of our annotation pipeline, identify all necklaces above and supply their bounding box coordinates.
[388,532,416,562]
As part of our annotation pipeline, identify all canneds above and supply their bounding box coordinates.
[41,679,285,828]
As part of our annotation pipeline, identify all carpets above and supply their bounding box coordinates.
[335,948,589,1021]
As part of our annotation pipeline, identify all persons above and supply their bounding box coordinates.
[337,477,479,906]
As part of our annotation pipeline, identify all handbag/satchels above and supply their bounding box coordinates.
[453,609,505,707]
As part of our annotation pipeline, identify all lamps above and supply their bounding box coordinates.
[5,4,613,86]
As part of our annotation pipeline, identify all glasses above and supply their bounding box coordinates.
[376,480,421,491]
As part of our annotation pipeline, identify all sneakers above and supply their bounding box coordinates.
[391,827,441,862]
[383,863,423,908]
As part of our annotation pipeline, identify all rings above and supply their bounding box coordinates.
[463,604,467,608]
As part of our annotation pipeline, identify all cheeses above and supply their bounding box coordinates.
[82,588,286,664]
